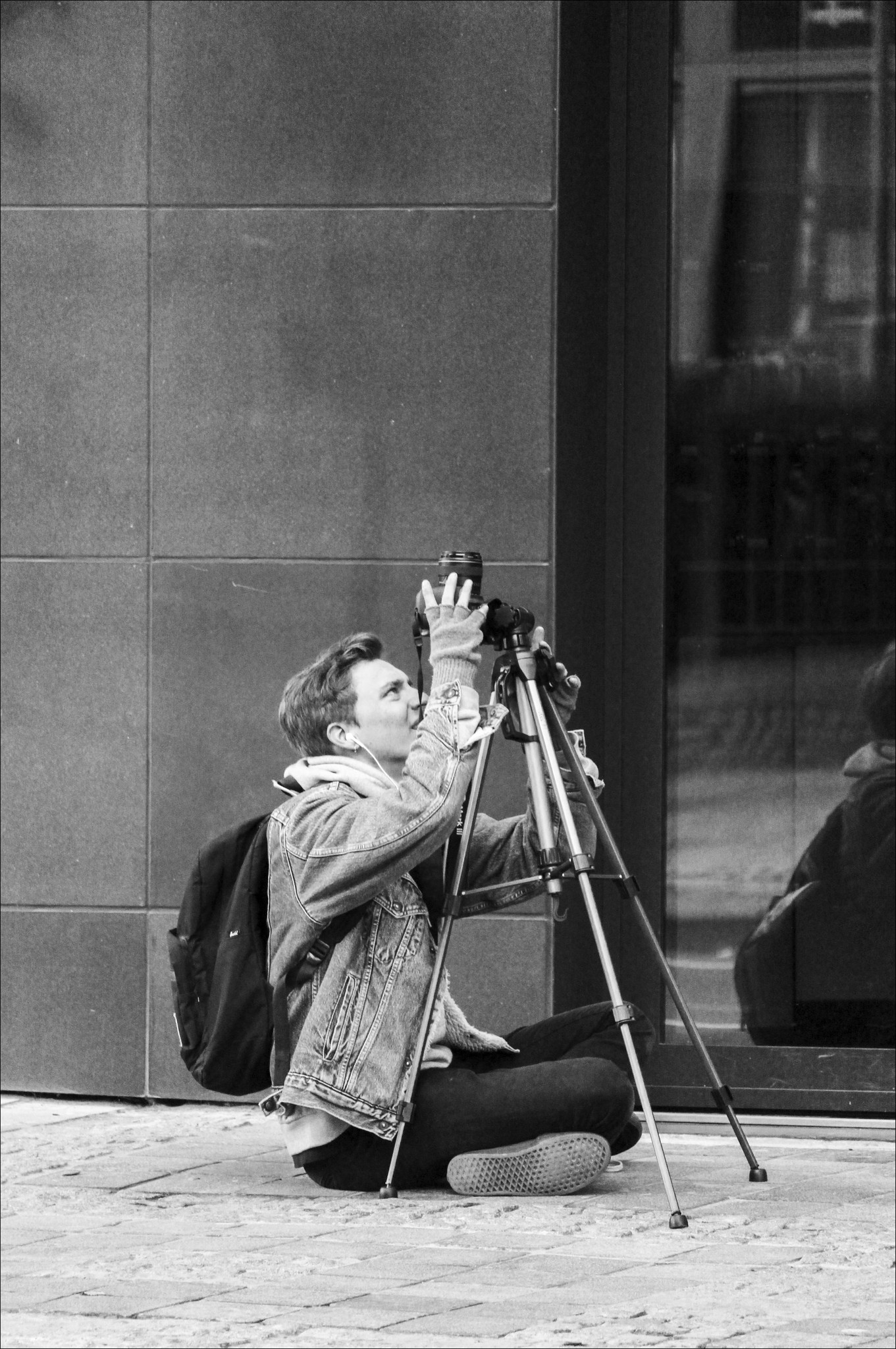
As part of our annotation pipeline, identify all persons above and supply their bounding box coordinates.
[821,637,895,1050]
[257,566,664,1196]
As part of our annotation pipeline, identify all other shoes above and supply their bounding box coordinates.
[447,1130,612,1198]
[611,1112,643,1155]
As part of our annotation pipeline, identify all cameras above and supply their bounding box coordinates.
[411,541,539,656]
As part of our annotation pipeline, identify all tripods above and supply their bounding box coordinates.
[374,629,771,1229]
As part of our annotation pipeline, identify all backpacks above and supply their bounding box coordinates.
[165,808,375,1096]
[731,793,847,1049]
